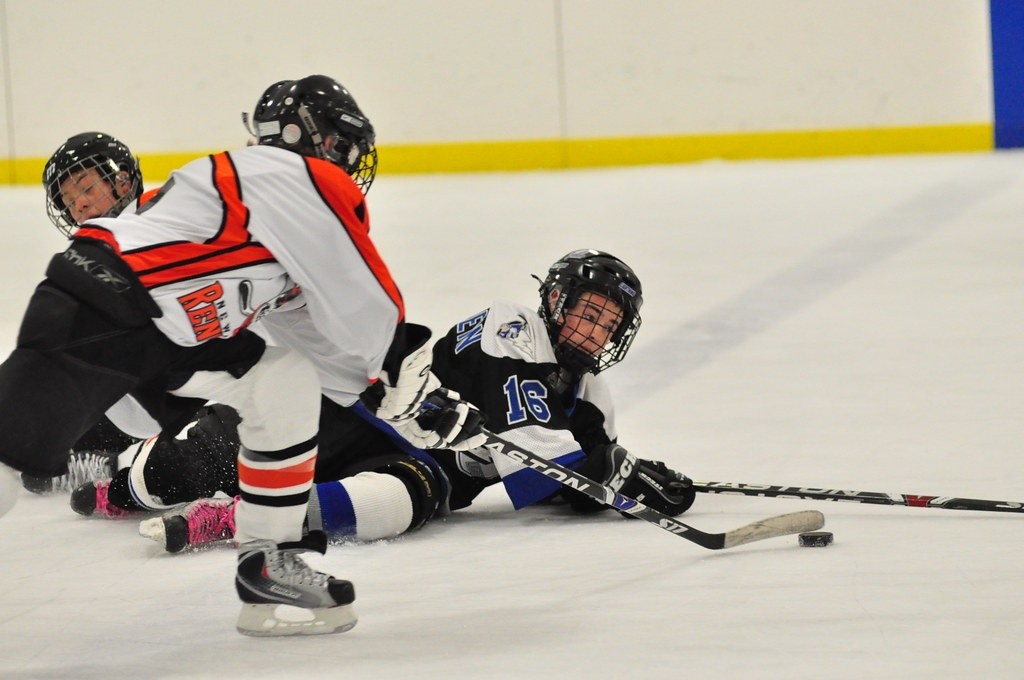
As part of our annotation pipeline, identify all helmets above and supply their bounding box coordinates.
[42,132,144,218]
[539,248,644,375]
[240,75,375,176]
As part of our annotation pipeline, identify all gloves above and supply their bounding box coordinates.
[358,321,491,451]
[602,442,696,520]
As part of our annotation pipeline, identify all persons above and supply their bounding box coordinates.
[72,247,697,554]
[1,74,405,638]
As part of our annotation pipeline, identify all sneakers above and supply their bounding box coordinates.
[70,473,138,520]
[233,531,358,635]
[21,449,122,497]
[138,490,241,554]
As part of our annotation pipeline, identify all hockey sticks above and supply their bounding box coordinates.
[690,479,1023,515]
[471,427,827,552]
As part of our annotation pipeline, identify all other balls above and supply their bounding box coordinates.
[796,531,835,549]
